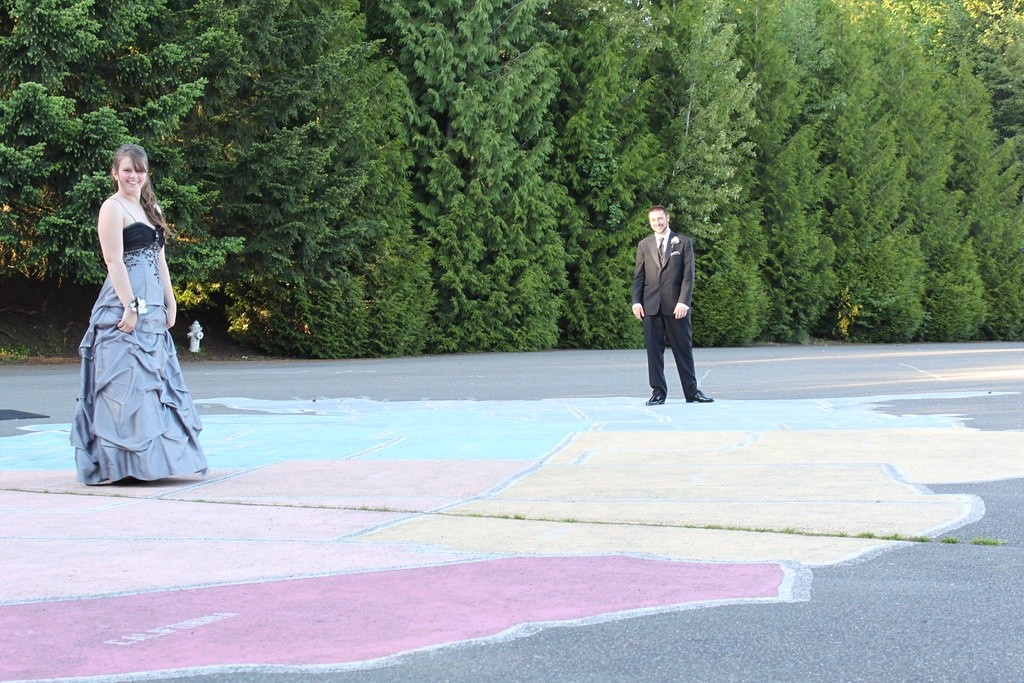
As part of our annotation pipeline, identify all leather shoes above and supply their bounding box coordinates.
[646,395,664,405]
[686,392,713,403]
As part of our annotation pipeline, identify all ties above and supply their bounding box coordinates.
[657,238,665,267]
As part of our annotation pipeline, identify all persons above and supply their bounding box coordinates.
[631,205,714,405]
[71,144,209,486]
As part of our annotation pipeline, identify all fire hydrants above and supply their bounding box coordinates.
[186,319,205,354]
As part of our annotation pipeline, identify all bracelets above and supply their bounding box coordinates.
[123,297,147,314]
